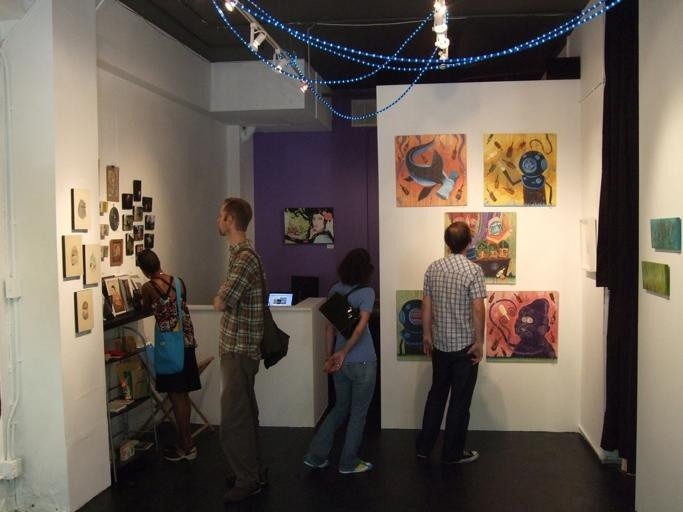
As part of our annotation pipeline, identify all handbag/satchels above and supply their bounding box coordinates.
[153,328,186,380]
[261,307,289,370]
[319,284,375,340]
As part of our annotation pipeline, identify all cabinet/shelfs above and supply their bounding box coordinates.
[102,307,161,482]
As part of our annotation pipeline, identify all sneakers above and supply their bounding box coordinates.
[440,450,479,465]
[163,444,198,462]
[304,458,329,470]
[339,460,373,474]
[222,483,262,504]
[225,469,267,486]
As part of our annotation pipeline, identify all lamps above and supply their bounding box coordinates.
[431,1,451,62]
[223,0,309,94]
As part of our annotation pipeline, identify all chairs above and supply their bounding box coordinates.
[135,341,215,442]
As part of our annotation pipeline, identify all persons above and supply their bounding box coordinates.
[308,208,334,243]
[415,221,486,465]
[214,197,290,503]
[110,286,122,310]
[304,248,377,475]
[136,250,202,462]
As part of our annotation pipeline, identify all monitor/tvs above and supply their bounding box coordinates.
[267,291,295,307]
